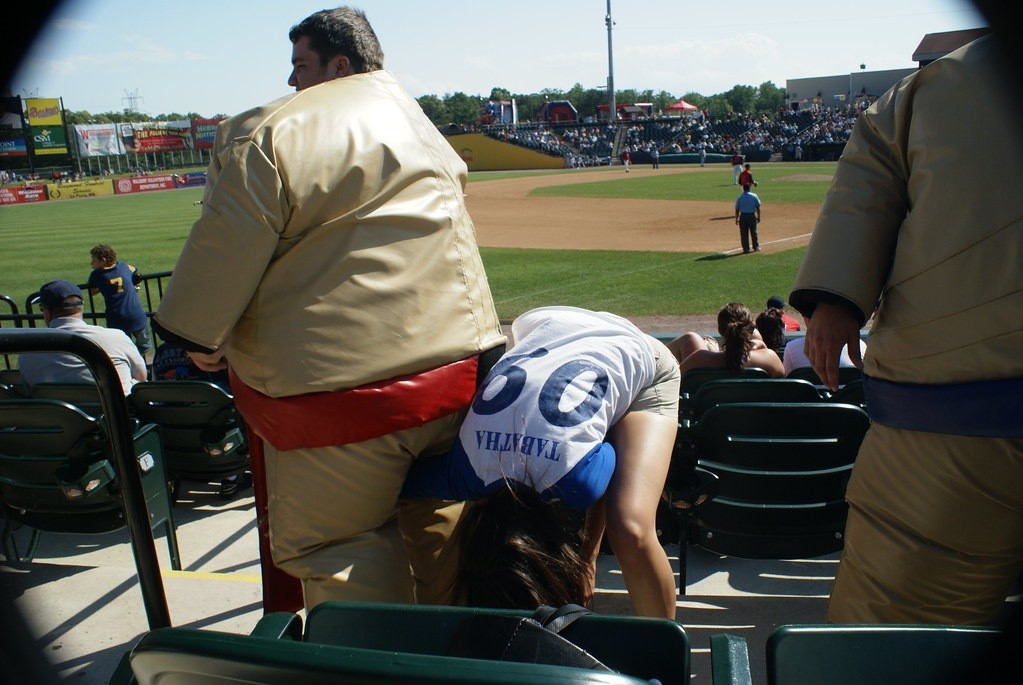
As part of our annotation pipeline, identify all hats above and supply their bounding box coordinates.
[745,164,751,168]
[30,280,84,307]
[767,296,788,310]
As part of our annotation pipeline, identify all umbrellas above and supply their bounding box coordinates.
[667,98,698,117]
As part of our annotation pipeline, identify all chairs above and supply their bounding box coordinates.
[0,365,1023,685]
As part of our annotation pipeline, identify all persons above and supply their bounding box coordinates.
[663,289,870,381]
[407,295,685,624]
[787,0,1023,625]
[738,164,758,193]
[16,282,172,425]
[732,151,744,184]
[476,98,877,173]
[151,4,505,610]
[86,242,151,357]
[735,183,761,254]
[149,305,252,499]
[1,163,164,183]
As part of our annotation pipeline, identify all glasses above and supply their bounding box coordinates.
[38,304,51,312]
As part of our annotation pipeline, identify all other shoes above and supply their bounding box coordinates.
[744,250,750,253]
[754,248,761,252]
